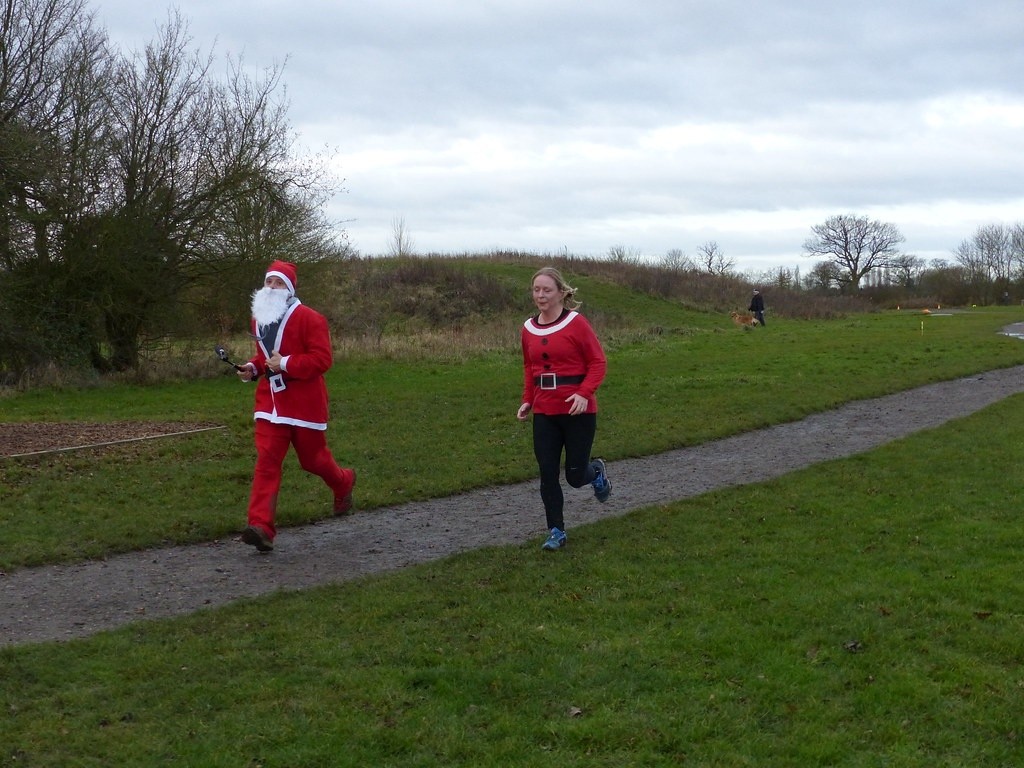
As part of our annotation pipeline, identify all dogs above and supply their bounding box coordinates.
[730,311,754,328]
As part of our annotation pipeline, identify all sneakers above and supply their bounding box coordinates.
[333,468,356,515]
[241,526,274,552]
[542,526,566,551]
[591,458,611,503]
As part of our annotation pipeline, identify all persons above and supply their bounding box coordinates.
[516,267,613,551]
[236,260,356,551]
[748,289,765,326]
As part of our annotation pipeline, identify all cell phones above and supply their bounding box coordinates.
[215,347,225,359]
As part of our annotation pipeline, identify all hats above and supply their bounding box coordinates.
[265,260,297,295]
[753,289,759,291]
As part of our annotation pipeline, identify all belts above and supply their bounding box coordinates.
[533,373,586,389]
